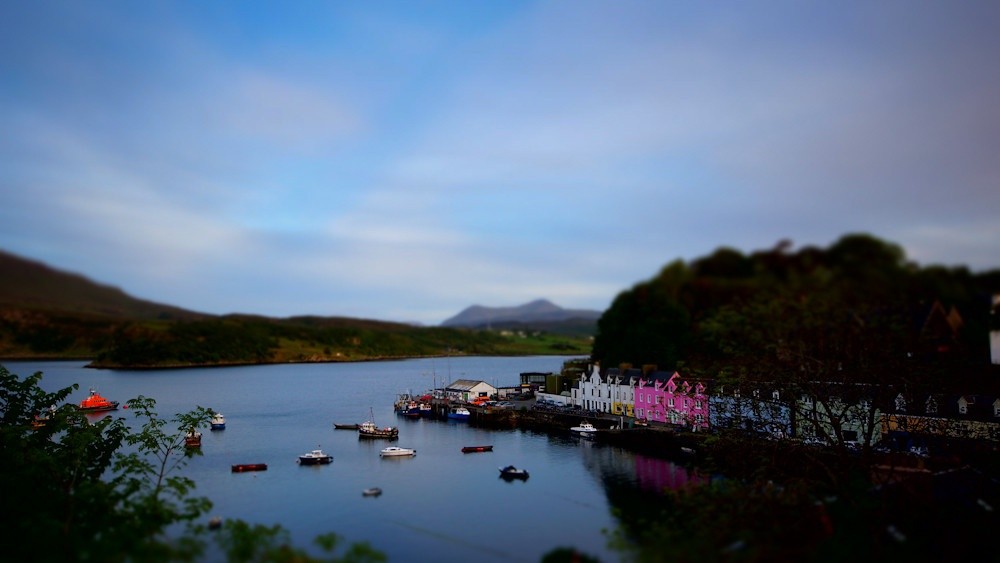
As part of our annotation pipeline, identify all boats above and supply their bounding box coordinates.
[569,422,596,435]
[231,463,267,472]
[393,388,470,421]
[356,421,399,440]
[75,391,119,411]
[461,444,493,453]
[334,423,359,430]
[29,417,54,431]
[498,466,529,481]
[210,413,225,430]
[298,450,332,465]
[185,432,201,449]
[380,447,415,457]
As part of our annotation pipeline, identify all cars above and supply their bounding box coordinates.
[531,401,603,418]
[472,396,514,408]
